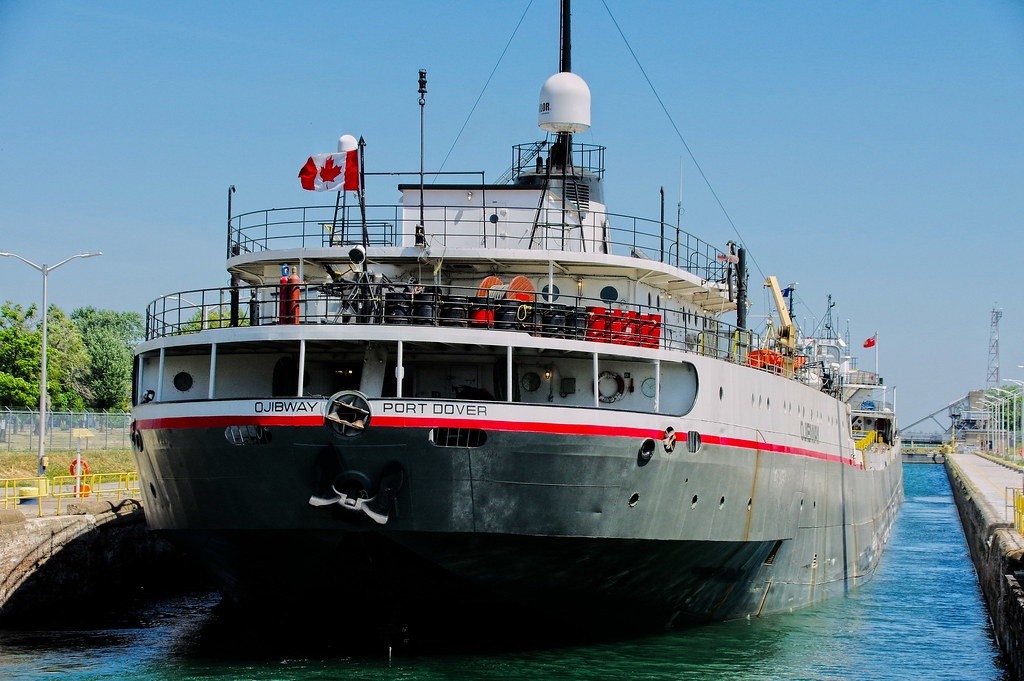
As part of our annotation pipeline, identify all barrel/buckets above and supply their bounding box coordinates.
[383,292,662,349]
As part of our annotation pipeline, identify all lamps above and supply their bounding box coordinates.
[141,390,155,404]
[350,244,365,272]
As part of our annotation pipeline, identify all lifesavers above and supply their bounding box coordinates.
[70,459,89,480]
[593,371,626,404]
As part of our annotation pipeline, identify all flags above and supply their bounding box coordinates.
[863,335,876,348]
[298,149,359,192]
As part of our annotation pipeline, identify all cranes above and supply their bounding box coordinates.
[986,306,1002,390]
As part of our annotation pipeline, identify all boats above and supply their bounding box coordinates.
[131,0,902,656]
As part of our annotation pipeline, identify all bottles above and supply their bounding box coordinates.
[279,264,300,324]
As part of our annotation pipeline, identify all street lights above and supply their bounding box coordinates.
[0,252,103,478]
[972,378,1024,464]
